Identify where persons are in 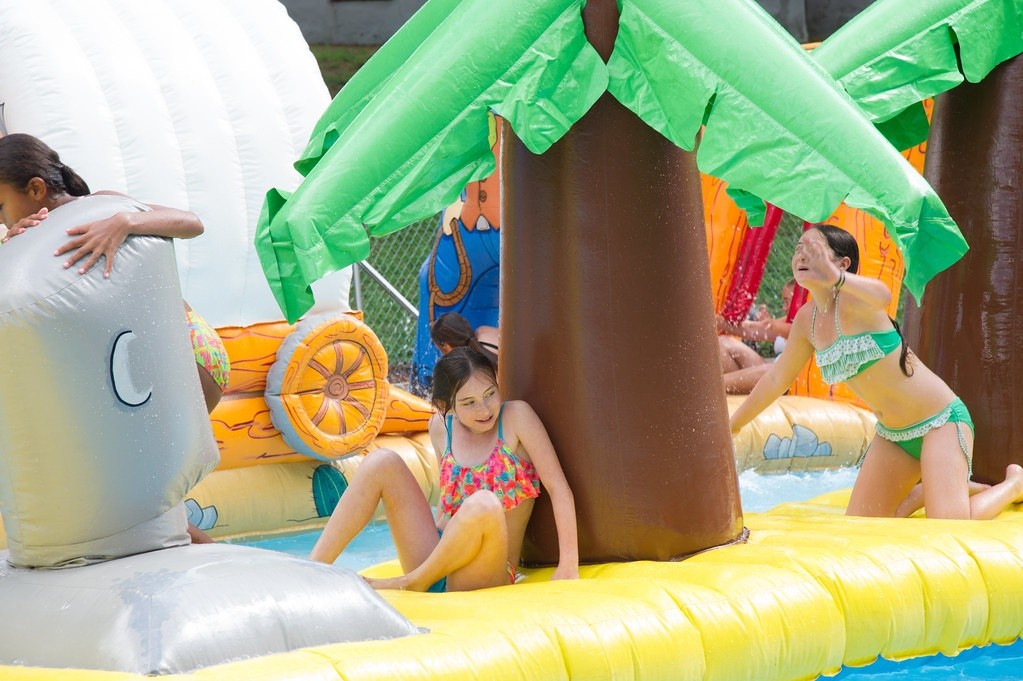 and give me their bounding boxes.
[0,133,230,544]
[308,346,578,593]
[431,313,497,380]
[716,280,796,393]
[728,224,1023,521]
